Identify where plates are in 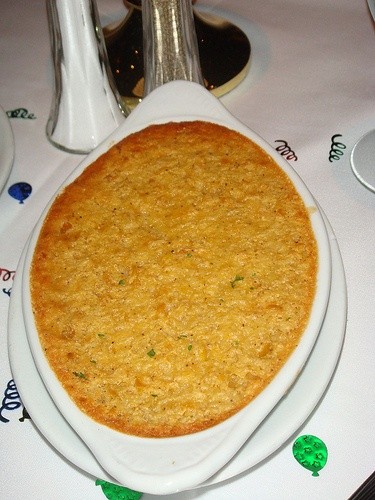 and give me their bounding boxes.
[8,80,348,495]
